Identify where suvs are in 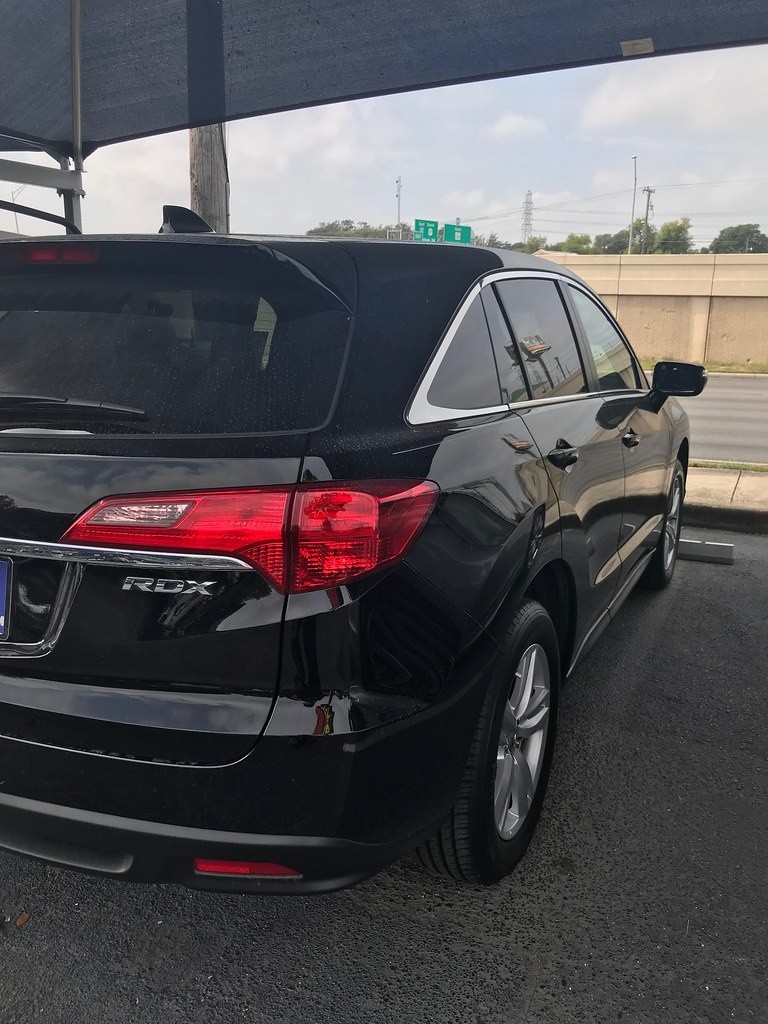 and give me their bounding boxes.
[0,203,713,893]
